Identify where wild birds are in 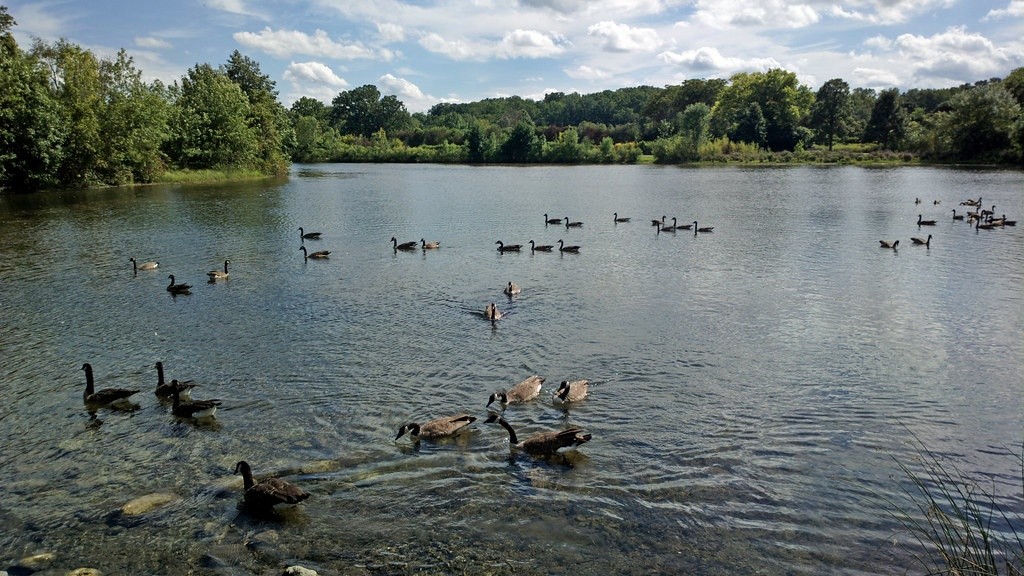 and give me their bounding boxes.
[613,212,716,236]
[207,260,232,279]
[878,196,1017,249]
[233,461,312,506]
[495,240,523,252]
[389,236,419,250]
[420,238,441,248]
[394,414,477,442]
[298,246,332,258]
[76,362,141,406]
[482,414,592,456]
[298,226,323,239]
[166,274,193,292]
[152,361,223,420]
[503,281,521,295]
[128,257,160,271]
[485,375,546,408]
[529,214,584,253]
[552,380,591,404]
[484,302,501,321]
